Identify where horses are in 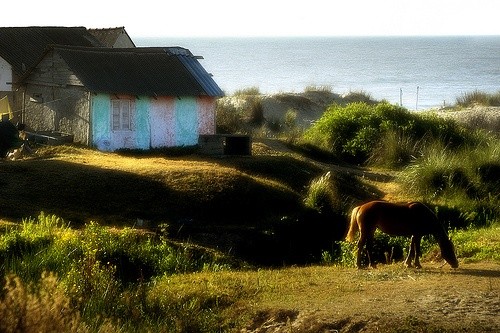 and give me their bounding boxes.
[346,200,458,269]
